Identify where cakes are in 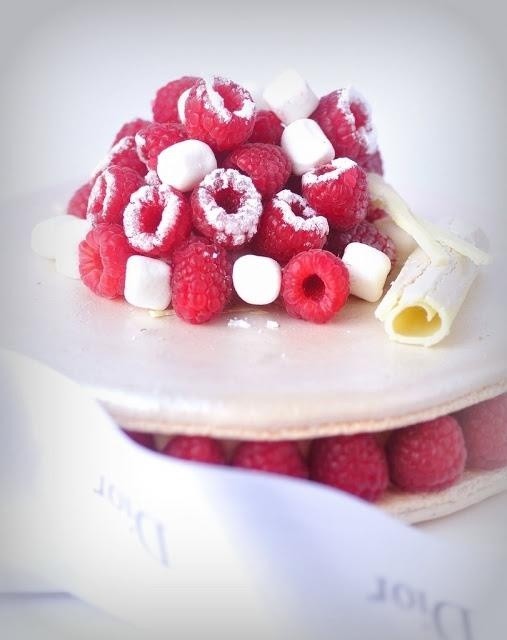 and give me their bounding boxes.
[26,70,507,528]
